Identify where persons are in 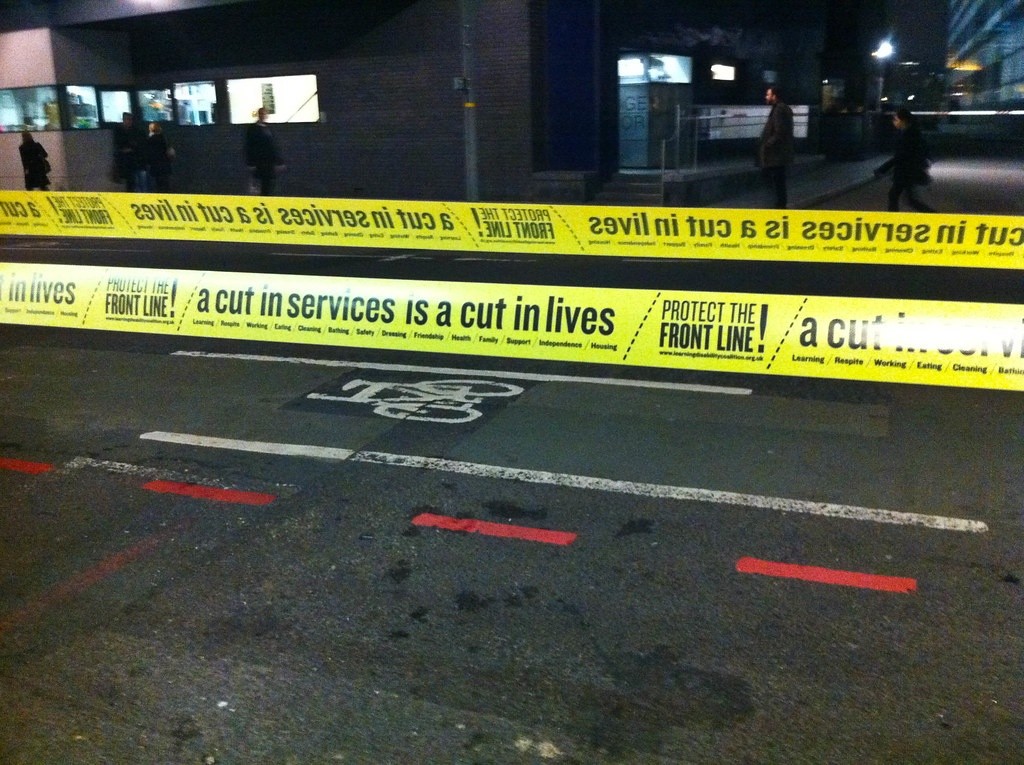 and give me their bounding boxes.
[246,108,276,195]
[759,84,794,208]
[112,113,168,192]
[18,131,51,190]
[873,108,936,213]
